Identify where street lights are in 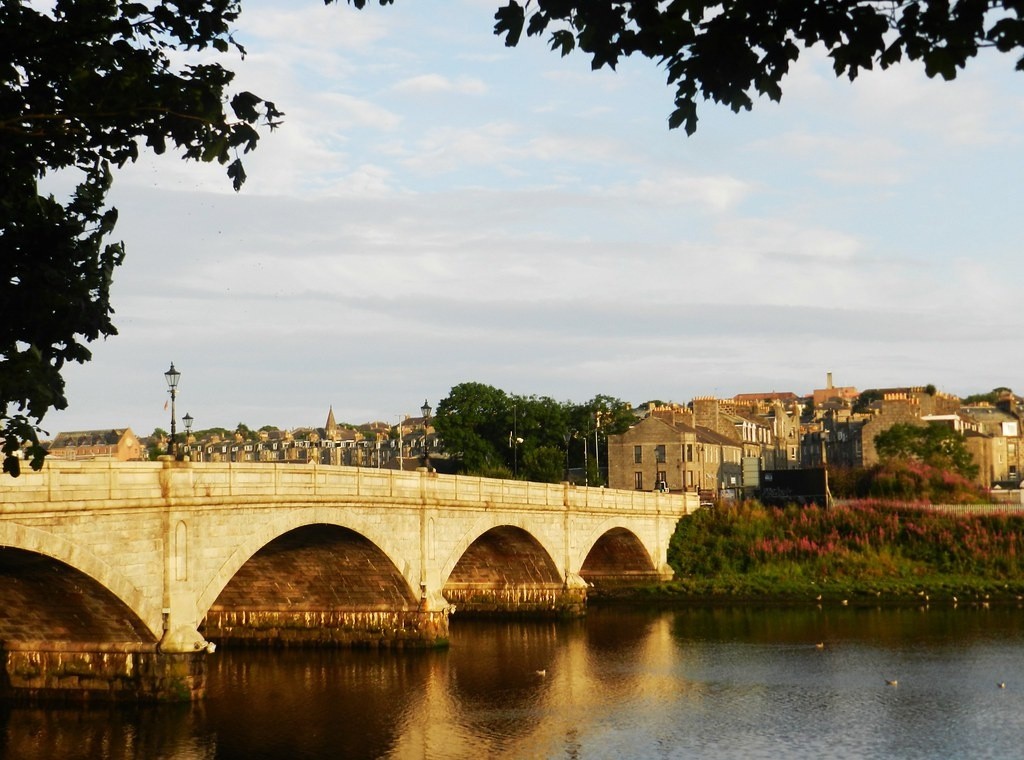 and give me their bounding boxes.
[421,399,432,470]
[164,361,181,462]
[182,412,195,461]
[393,414,408,469]
